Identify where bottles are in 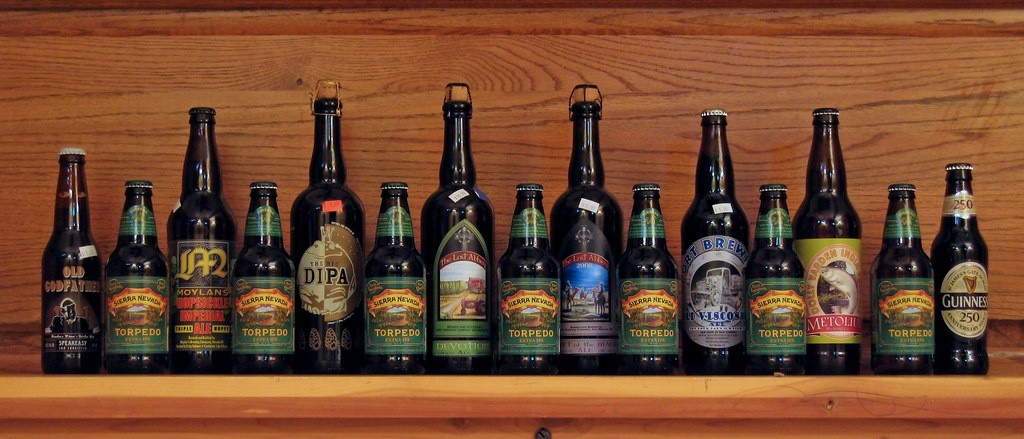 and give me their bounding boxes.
[166,107,235,376]
[791,107,864,377]
[104,180,167,375]
[40,148,103,377]
[550,85,617,377]
[746,184,806,376]
[496,183,561,377]
[290,81,364,376]
[364,182,426,375]
[869,184,937,376]
[230,182,293,375]
[930,161,990,377]
[618,184,680,376]
[420,83,496,376]
[681,110,748,377]
